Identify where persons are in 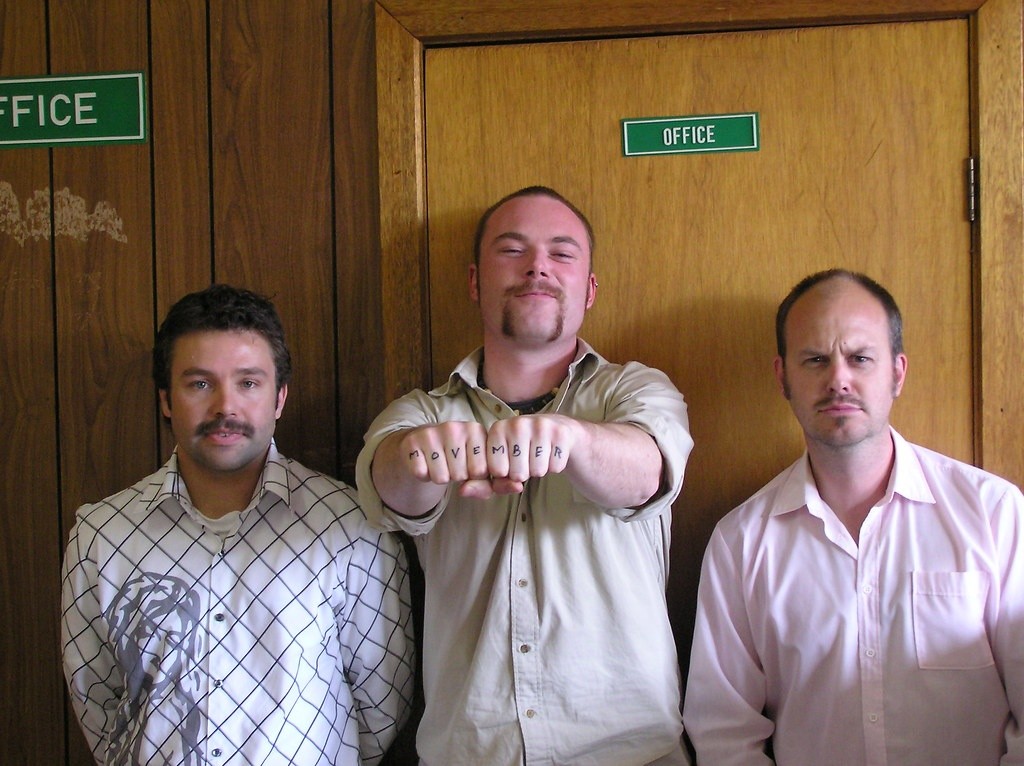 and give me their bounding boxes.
[679,267,1024,766]
[354,185,695,766]
[60,283,418,766]
